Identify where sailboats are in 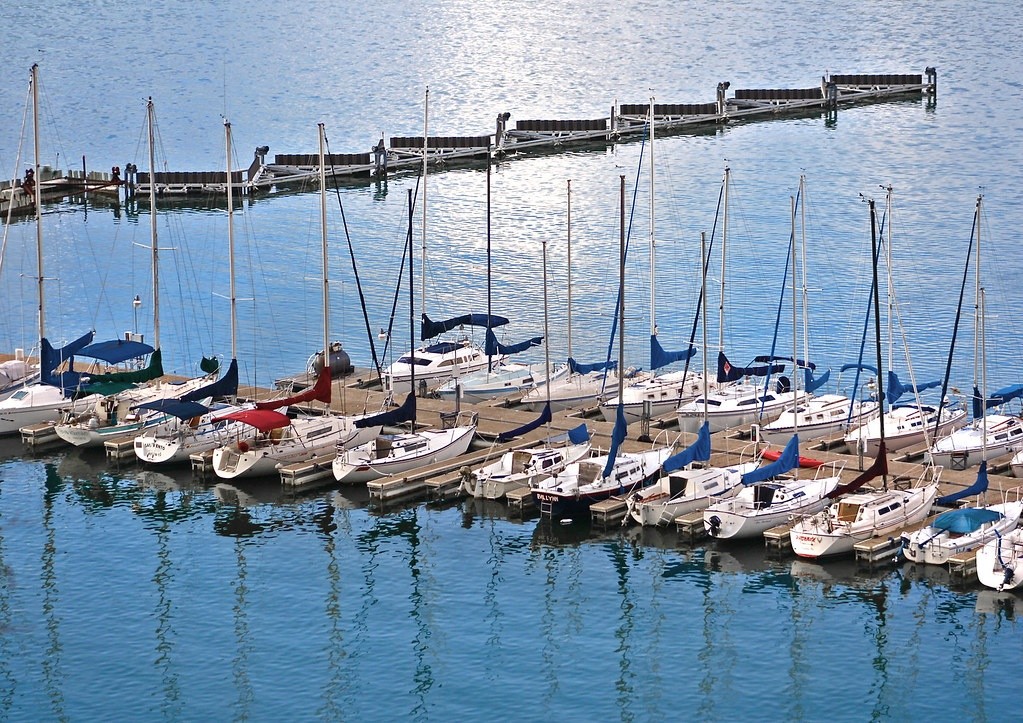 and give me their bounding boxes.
[0,56,1022,596]
